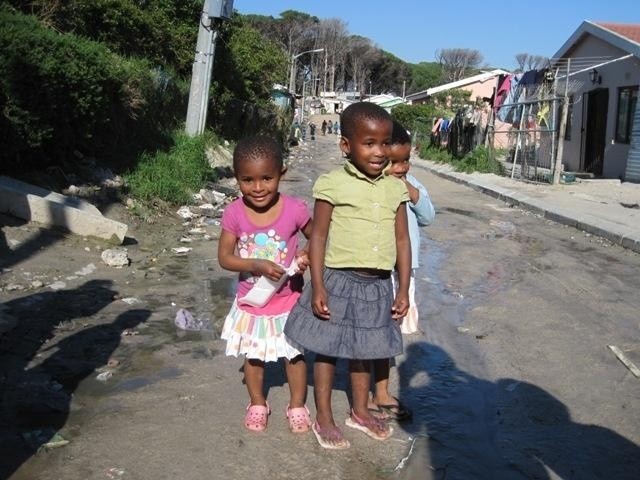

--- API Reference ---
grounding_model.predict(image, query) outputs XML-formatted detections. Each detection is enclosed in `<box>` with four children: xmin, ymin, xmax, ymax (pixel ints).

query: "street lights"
<box><xmin>368</xmin><ymin>81</ymin><xmax>372</xmax><ymax>102</ymax></box>
<box><xmin>301</xmin><ymin>78</ymin><xmax>321</xmax><ymax>123</ymax></box>
<box><xmin>289</xmin><ymin>49</ymin><xmax>325</xmax><ymax>106</ymax></box>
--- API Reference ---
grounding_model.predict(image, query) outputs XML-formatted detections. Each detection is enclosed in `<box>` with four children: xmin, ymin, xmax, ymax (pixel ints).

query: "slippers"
<box><xmin>369</xmin><ymin>395</ymin><xmax>412</xmax><ymax>422</ymax></box>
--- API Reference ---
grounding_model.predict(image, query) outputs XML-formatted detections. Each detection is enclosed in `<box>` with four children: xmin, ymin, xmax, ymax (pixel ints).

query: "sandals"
<box><xmin>244</xmin><ymin>400</ymin><xmax>269</xmax><ymax>431</ymax></box>
<box><xmin>345</xmin><ymin>408</ymin><xmax>393</xmax><ymax>441</ymax></box>
<box><xmin>312</xmin><ymin>420</ymin><xmax>350</xmax><ymax>449</ymax></box>
<box><xmin>286</xmin><ymin>404</ymin><xmax>310</xmax><ymax>433</ymax></box>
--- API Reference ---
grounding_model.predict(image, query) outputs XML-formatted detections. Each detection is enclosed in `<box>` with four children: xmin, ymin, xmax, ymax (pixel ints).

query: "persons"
<box><xmin>362</xmin><ymin>118</ymin><xmax>435</xmax><ymax>424</ymax></box>
<box><xmin>281</xmin><ymin>102</ymin><xmax>412</xmax><ymax>449</ymax></box>
<box><xmin>216</xmin><ymin>135</ymin><xmax>316</xmax><ymax>436</ymax></box>
<box><xmin>289</xmin><ymin>119</ymin><xmax>338</xmax><ymax>145</ymax></box>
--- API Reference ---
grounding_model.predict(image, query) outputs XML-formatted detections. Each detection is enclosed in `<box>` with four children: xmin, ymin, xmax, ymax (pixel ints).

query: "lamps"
<box><xmin>588</xmin><ymin>68</ymin><xmax>602</xmax><ymax>85</ymax></box>
